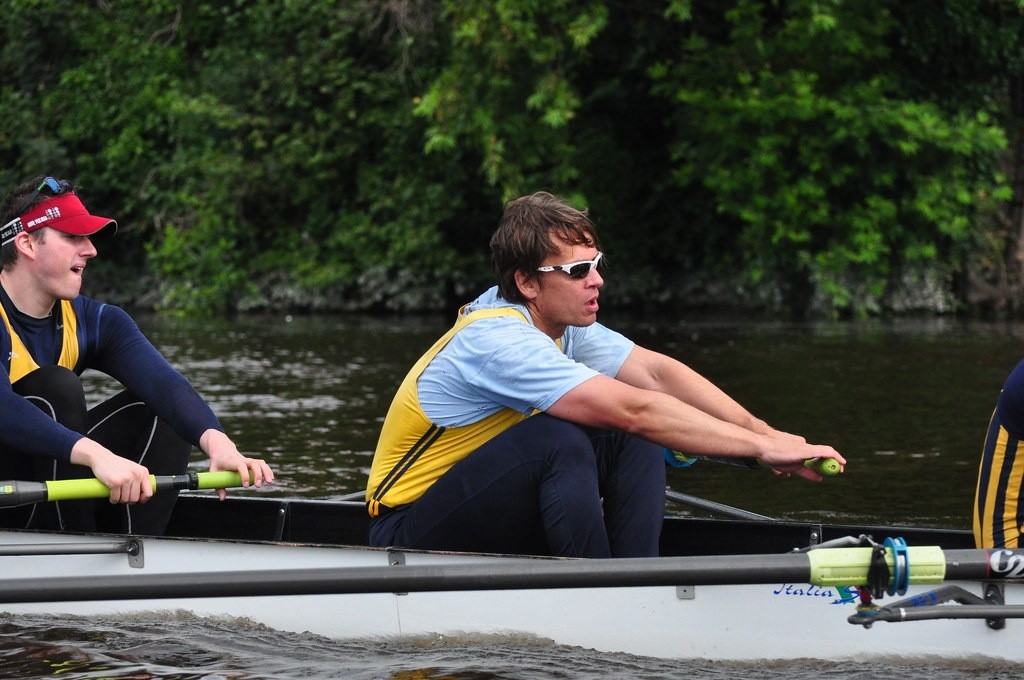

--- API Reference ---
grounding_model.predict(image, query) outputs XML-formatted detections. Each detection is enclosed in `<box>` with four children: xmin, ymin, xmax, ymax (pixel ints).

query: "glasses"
<box><xmin>531</xmin><ymin>251</ymin><xmax>603</xmax><ymax>280</ymax></box>
<box><xmin>9</xmin><ymin>176</ymin><xmax>74</xmax><ymax>223</ymax></box>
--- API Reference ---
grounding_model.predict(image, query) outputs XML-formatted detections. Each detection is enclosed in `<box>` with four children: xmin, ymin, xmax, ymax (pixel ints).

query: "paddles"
<box><xmin>684</xmin><ymin>444</ymin><xmax>842</xmax><ymax>480</ymax></box>
<box><xmin>2</xmin><ymin>533</ymin><xmax>1024</xmax><ymax>612</ymax></box>
<box><xmin>0</xmin><ymin>468</ymin><xmax>268</xmax><ymax>513</ymax></box>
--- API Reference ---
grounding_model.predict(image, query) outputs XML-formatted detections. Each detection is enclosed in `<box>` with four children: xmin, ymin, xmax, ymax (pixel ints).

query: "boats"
<box><xmin>0</xmin><ymin>491</ymin><xmax>1024</xmax><ymax>667</ymax></box>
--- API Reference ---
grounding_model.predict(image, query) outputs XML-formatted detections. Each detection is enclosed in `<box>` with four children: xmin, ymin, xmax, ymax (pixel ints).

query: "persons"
<box><xmin>973</xmin><ymin>361</ymin><xmax>1024</xmax><ymax>549</ymax></box>
<box><xmin>364</xmin><ymin>191</ymin><xmax>847</xmax><ymax>559</ymax></box>
<box><xmin>0</xmin><ymin>174</ymin><xmax>275</xmax><ymax>536</ymax></box>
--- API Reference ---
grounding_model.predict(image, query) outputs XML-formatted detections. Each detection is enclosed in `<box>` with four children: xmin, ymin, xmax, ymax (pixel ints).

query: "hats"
<box><xmin>0</xmin><ymin>192</ymin><xmax>118</xmax><ymax>247</ymax></box>
